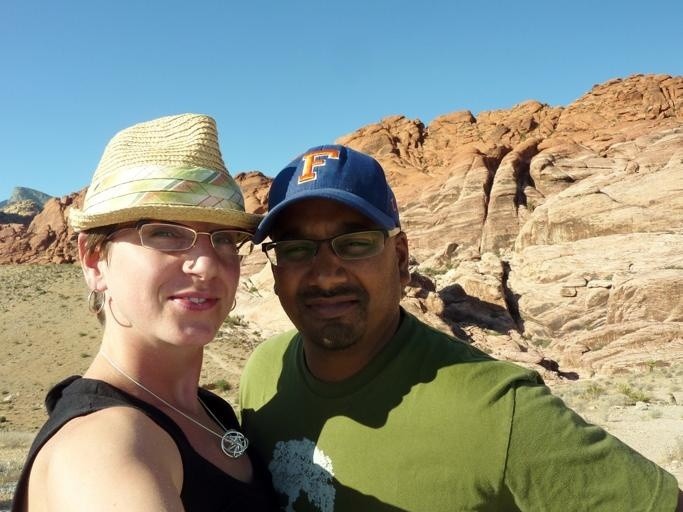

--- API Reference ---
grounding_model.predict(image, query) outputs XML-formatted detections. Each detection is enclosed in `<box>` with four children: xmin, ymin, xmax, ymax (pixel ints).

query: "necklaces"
<box><xmin>99</xmin><ymin>349</ymin><xmax>250</xmax><ymax>460</ymax></box>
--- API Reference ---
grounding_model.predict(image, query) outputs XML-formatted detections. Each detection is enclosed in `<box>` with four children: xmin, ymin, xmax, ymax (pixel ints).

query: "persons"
<box><xmin>10</xmin><ymin>113</ymin><xmax>289</xmax><ymax>511</ymax></box>
<box><xmin>240</xmin><ymin>145</ymin><xmax>683</xmax><ymax>512</ymax></box>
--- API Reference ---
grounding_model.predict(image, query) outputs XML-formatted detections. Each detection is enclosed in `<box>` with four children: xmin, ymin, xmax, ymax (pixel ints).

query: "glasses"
<box><xmin>104</xmin><ymin>223</ymin><xmax>255</xmax><ymax>256</ymax></box>
<box><xmin>262</xmin><ymin>227</ymin><xmax>401</xmax><ymax>270</ymax></box>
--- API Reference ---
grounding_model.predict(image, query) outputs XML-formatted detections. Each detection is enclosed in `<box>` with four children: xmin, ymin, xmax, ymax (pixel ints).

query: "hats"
<box><xmin>68</xmin><ymin>113</ymin><xmax>265</xmax><ymax>230</ymax></box>
<box><xmin>254</xmin><ymin>144</ymin><xmax>401</xmax><ymax>243</ymax></box>
